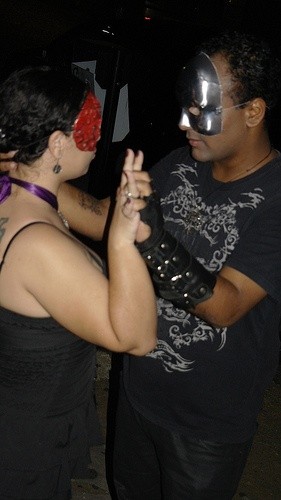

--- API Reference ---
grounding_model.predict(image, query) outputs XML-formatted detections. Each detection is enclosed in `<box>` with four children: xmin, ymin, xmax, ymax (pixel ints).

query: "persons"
<box><xmin>1</xmin><ymin>28</ymin><xmax>281</xmax><ymax>500</ymax></box>
<box><xmin>1</xmin><ymin>65</ymin><xmax>158</xmax><ymax>500</ymax></box>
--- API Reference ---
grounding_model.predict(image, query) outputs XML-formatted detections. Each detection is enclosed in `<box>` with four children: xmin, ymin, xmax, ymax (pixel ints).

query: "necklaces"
<box><xmin>219</xmin><ymin>145</ymin><xmax>273</xmax><ymax>182</ymax></box>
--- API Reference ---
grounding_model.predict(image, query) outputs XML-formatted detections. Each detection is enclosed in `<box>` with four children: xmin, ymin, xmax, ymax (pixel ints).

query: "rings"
<box><xmin>127</xmin><ymin>193</ymin><xmax>136</xmax><ymax>207</ymax></box>
